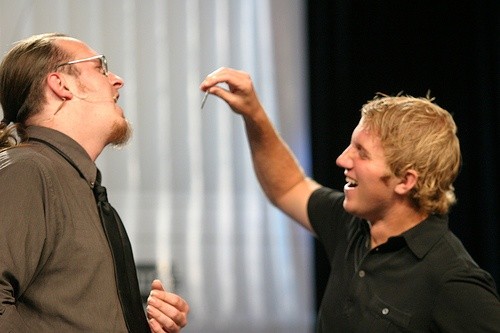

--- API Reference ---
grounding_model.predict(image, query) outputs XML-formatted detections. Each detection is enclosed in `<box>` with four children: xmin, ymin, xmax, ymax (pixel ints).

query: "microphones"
<box><xmin>82</xmin><ymin>96</ymin><xmax>116</xmax><ymax>103</ymax></box>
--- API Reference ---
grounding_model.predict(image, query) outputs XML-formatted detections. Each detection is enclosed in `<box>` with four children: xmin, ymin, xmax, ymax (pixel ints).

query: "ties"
<box><xmin>92</xmin><ymin>168</ymin><xmax>151</xmax><ymax>333</ymax></box>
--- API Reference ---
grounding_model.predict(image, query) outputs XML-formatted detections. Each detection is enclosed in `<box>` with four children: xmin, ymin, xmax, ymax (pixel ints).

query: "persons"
<box><xmin>200</xmin><ymin>67</ymin><xmax>500</xmax><ymax>333</ymax></box>
<box><xmin>0</xmin><ymin>33</ymin><xmax>189</xmax><ymax>333</ymax></box>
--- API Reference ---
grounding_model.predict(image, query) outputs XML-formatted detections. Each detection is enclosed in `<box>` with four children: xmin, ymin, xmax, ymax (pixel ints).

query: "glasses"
<box><xmin>55</xmin><ymin>54</ymin><xmax>108</xmax><ymax>77</ymax></box>
